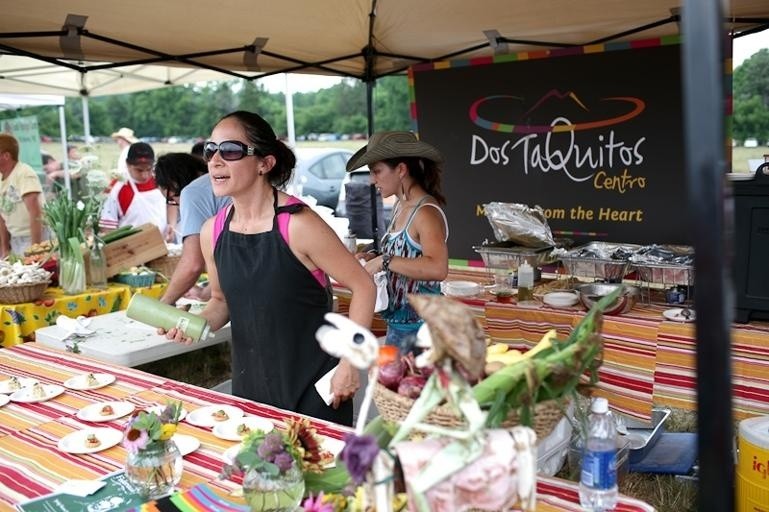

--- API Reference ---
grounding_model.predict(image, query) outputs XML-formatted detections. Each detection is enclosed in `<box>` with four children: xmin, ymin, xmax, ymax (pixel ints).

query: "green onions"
<box><xmin>39</xmin><ymin>179</ymin><xmax>106</xmax><ymax>294</ymax></box>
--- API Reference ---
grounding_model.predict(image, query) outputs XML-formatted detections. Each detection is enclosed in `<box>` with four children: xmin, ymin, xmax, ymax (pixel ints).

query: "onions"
<box><xmin>375</xmin><ymin>358</ymin><xmax>434</xmax><ymax>398</ymax></box>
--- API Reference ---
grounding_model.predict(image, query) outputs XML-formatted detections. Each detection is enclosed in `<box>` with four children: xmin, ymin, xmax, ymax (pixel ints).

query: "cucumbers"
<box><xmin>102</xmin><ymin>223</ymin><xmax>143</xmax><ymax>246</ymax></box>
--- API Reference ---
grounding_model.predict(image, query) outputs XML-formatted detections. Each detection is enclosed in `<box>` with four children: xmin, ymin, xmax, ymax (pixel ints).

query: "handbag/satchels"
<box><xmin>358</xmin><ymin>258</ymin><xmax>389</xmax><ymax>314</ymax></box>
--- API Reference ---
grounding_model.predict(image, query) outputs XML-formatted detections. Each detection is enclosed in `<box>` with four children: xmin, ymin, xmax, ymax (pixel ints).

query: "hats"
<box><xmin>112</xmin><ymin>127</ymin><xmax>140</xmax><ymax>144</ymax></box>
<box><xmin>346</xmin><ymin>131</ymin><xmax>445</xmax><ymax>172</ymax></box>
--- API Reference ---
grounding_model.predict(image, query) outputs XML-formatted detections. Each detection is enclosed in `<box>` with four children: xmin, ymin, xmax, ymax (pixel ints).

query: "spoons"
<box><xmin>682</xmin><ymin>307</ymin><xmax>690</xmax><ymax>322</ymax></box>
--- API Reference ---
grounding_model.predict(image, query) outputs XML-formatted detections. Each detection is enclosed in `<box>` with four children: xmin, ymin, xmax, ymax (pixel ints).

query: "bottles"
<box><xmin>577</xmin><ymin>394</ymin><xmax>619</xmax><ymax>510</ymax></box>
<box><xmin>124</xmin><ymin>291</ymin><xmax>216</xmax><ymax>347</ymax></box>
<box><xmin>126</xmin><ymin>441</ymin><xmax>184</xmax><ymax>498</ymax></box>
<box><xmin>516</xmin><ymin>259</ymin><xmax>534</xmax><ymax>304</ymax></box>
<box><xmin>242</xmin><ymin>465</ymin><xmax>305</xmax><ymax>512</ymax></box>
<box><xmin>343</xmin><ymin>234</ymin><xmax>357</xmax><ymax>254</ymax></box>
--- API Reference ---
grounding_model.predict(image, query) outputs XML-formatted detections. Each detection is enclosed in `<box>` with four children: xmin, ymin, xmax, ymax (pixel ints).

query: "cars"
<box><xmin>290</xmin><ymin>146</ymin><xmax>370</xmax><ymax>210</ymax></box>
<box><xmin>40</xmin><ymin>133</ymin><xmax>203</xmax><ymax>145</ymax></box>
<box><xmin>743</xmin><ymin>137</ymin><xmax>759</xmax><ymax>148</ymax></box>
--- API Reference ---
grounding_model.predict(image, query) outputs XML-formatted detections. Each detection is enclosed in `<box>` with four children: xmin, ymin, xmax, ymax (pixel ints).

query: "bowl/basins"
<box><xmin>574</xmin><ymin>283</ymin><xmax>642</xmax><ymax>317</ymax></box>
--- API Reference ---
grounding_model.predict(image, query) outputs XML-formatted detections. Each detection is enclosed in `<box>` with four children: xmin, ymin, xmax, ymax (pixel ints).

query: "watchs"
<box><xmin>380</xmin><ymin>254</ymin><xmax>391</xmax><ymax>270</ymax></box>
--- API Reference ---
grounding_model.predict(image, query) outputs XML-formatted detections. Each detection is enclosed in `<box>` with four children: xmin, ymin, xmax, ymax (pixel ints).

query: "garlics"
<box><xmin>0</xmin><ymin>259</ymin><xmax>50</xmax><ymax>287</ymax></box>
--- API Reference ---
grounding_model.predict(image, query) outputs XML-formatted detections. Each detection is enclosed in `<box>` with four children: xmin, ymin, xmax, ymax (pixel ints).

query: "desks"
<box><xmin>0</xmin><ymin>258</ymin><xmax>768</xmax><ymax>512</ymax></box>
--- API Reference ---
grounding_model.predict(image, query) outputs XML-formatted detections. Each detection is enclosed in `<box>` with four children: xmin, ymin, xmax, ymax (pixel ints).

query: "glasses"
<box><xmin>202</xmin><ymin>140</ymin><xmax>258</xmax><ymax>161</ymax></box>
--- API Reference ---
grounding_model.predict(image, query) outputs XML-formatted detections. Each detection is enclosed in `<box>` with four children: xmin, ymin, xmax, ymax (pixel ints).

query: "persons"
<box><xmin>158</xmin><ymin>110</ymin><xmax>378</xmax><ymax>428</ymax></box>
<box><xmin>358</xmin><ymin>128</ymin><xmax>449</xmax><ymax>351</ymax></box>
<box><xmin>37</xmin><ymin>127</ymin><xmax>167</xmax><ymax>232</ymax></box>
<box><xmin>150</xmin><ymin>153</ymin><xmax>233</xmax><ymax>336</ymax></box>
<box><xmin>1</xmin><ymin>134</ymin><xmax>59</xmax><ymax>258</ymax></box>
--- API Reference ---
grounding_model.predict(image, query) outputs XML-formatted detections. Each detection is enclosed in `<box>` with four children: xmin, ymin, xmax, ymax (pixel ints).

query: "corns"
<box><xmin>470</xmin><ymin>287</ymin><xmax>625</xmax><ymax>427</ymax></box>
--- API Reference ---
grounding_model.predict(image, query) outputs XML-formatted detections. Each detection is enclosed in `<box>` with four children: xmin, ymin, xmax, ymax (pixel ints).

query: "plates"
<box><xmin>186</xmin><ymin>404</ymin><xmax>246</xmax><ymax>427</ymax></box>
<box><xmin>9</xmin><ymin>384</ymin><xmax>65</xmax><ymax>403</ymax></box>
<box><xmin>76</xmin><ymin>401</ymin><xmax>135</xmax><ymax>423</ymax></box>
<box><xmin>0</xmin><ymin>394</ymin><xmax>9</xmax><ymax>407</ymax></box>
<box><xmin>213</xmin><ymin>417</ymin><xmax>275</xmax><ymax>441</ymax></box>
<box><xmin>725</xmin><ymin>172</ymin><xmax>754</xmax><ymax>181</ymax></box>
<box><xmin>543</xmin><ymin>291</ymin><xmax>580</xmax><ymax>309</ymax></box>
<box><xmin>171</xmin><ymin>434</ymin><xmax>201</xmax><ymax>456</ymax></box>
<box><xmin>64</xmin><ymin>373</ymin><xmax>116</xmax><ymax>391</ymax></box>
<box><xmin>145</xmin><ymin>404</ymin><xmax>186</xmax><ymax>422</ymax></box>
<box><xmin>662</xmin><ymin>307</ymin><xmax>698</xmax><ymax>323</ymax></box>
<box><xmin>489</xmin><ymin>288</ymin><xmax>517</xmax><ymax>296</ymax></box>
<box><xmin>443</xmin><ymin>278</ymin><xmax>481</xmax><ymax>298</ymax></box>
<box><xmin>288</xmin><ymin>434</ymin><xmax>346</xmax><ymax>471</ymax></box>
<box><xmin>0</xmin><ymin>377</ymin><xmax>40</xmax><ymax>394</ymax></box>
<box><xmin>220</xmin><ymin>441</ymin><xmax>247</xmax><ymax>466</ymax></box>
<box><xmin>57</xmin><ymin>427</ymin><xmax>124</xmax><ymax>455</ymax></box>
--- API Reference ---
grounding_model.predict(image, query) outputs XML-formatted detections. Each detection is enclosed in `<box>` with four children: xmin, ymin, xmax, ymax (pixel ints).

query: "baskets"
<box><xmin>474</xmin><ymin>240</ymin><xmax>696</xmax><ymax>307</ymax></box>
<box><xmin>0</xmin><ymin>280</ymin><xmax>52</xmax><ymax>304</ymax></box>
<box><xmin>369</xmin><ymin>372</ymin><xmax>570</xmax><ymax>444</ymax></box>
<box><xmin>114</xmin><ymin>248</ymin><xmax>182</xmax><ymax>287</ymax></box>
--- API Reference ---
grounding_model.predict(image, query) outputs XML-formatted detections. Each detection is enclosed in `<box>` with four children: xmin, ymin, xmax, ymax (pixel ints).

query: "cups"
<box><xmin>747</xmin><ymin>158</ymin><xmax>768</xmax><ymax>173</ymax></box>
<box><xmin>493</xmin><ymin>272</ymin><xmax>514</xmax><ymax>304</ymax></box>
